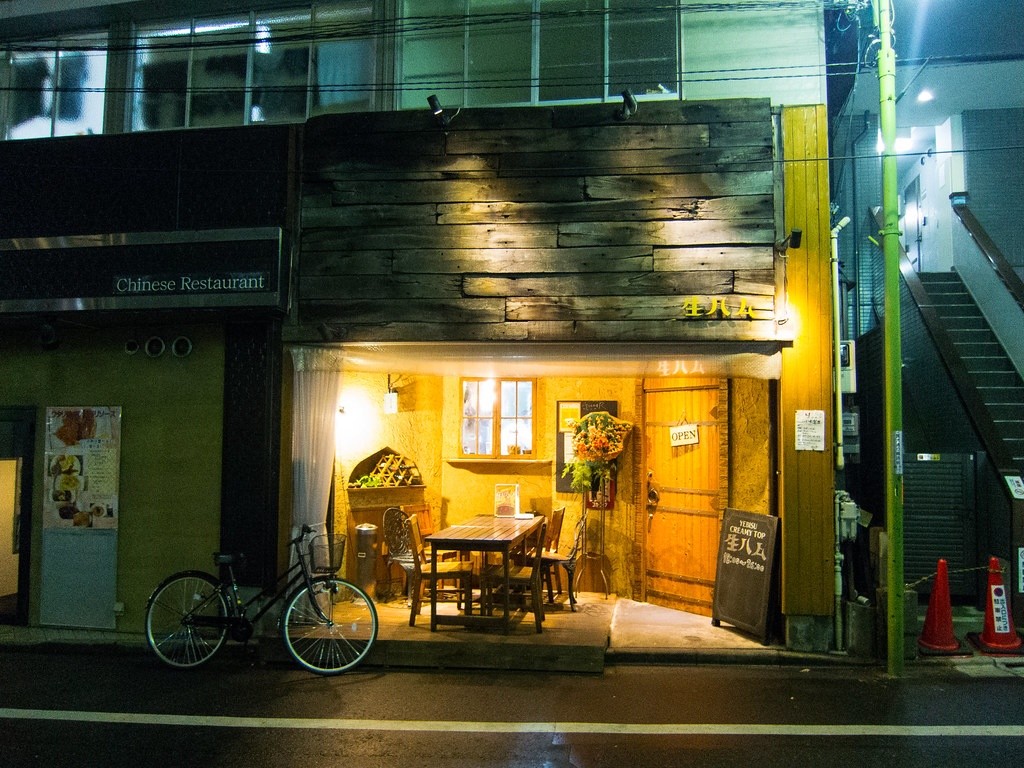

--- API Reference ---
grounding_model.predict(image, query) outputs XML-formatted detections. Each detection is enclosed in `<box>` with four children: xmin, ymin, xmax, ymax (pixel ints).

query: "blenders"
<box><xmin>480</xmin><ymin>421</ymin><xmax>491</xmax><ymax>454</ymax></box>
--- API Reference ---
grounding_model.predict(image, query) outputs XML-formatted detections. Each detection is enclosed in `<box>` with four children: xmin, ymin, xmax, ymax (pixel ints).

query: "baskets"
<box><xmin>308</xmin><ymin>534</ymin><xmax>347</xmax><ymax>574</ymax></box>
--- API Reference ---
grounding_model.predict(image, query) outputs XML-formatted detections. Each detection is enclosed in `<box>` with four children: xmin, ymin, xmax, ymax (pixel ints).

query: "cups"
<box><xmin>523</xmin><ymin>449</ymin><xmax>531</xmax><ymax>454</ymax></box>
<box><xmin>858</xmin><ymin>596</ymin><xmax>871</xmax><ymax>606</ymax></box>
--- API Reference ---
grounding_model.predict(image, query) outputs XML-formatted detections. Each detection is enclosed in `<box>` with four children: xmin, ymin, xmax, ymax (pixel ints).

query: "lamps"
<box><xmin>776</xmin><ymin>227</ymin><xmax>802</xmax><ymax>257</ymax></box>
<box><xmin>427</xmin><ymin>93</ymin><xmax>452</xmax><ymax>126</ymax></box>
<box><xmin>615</xmin><ymin>88</ymin><xmax>638</xmax><ymax>120</ymax></box>
<box><xmin>384</xmin><ymin>386</ymin><xmax>399</xmax><ymax>416</ymax></box>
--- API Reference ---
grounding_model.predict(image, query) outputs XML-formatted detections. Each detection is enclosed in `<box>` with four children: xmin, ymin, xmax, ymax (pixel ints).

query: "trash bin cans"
<box><xmin>351</xmin><ymin>523</ymin><xmax>380</xmax><ymax>604</ymax></box>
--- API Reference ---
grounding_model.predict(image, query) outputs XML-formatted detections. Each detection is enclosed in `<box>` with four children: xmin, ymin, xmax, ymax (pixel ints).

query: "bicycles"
<box><xmin>144</xmin><ymin>524</ymin><xmax>381</xmax><ymax>677</ymax></box>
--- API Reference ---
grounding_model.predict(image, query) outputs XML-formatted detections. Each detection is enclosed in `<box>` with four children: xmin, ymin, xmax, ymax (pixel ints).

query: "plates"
<box><xmin>53</xmin><ymin>501</ymin><xmax>83</xmax><ymax>522</ymax></box>
<box><xmin>54</xmin><ymin>474</ymin><xmax>86</xmax><ymax>490</ymax></box>
<box><xmin>74</xmin><ymin>512</ymin><xmax>92</xmax><ymax>528</ymax></box>
<box><xmin>91</xmin><ymin>502</ymin><xmax>107</xmax><ymax>518</ymax></box>
<box><xmin>48</xmin><ymin>488</ymin><xmax>76</xmax><ymax>502</ymax></box>
<box><xmin>50</xmin><ymin>454</ymin><xmax>81</xmax><ymax>476</ymax></box>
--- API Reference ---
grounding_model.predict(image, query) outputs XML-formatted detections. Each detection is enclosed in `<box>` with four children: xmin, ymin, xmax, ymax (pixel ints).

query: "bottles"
<box><xmin>507</xmin><ymin>431</ymin><xmax>521</xmax><ymax>454</ymax></box>
<box><xmin>379</xmin><ymin>462</ymin><xmax>414</xmax><ymax>486</ymax></box>
<box><xmin>464</xmin><ymin>446</ymin><xmax>470</xmax><ymax>455</ymax></box>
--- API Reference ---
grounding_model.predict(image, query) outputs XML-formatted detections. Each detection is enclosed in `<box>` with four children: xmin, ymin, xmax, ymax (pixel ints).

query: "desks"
<box><xmin>424</xmin><ymin>513</ymin><xmax>545</xmax><ymax>632</ymax></box>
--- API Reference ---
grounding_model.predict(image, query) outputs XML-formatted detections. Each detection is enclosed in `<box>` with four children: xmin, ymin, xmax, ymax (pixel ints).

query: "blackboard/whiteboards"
<box><xmin>713</xmin><ymin>507</ymin><xmax>782</xmax><ymax>637</ymax></box>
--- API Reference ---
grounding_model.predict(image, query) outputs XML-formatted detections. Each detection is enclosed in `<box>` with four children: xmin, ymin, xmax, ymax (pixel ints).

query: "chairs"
<box><xmin>405</xmin><ymin>513</ymin><xmax>475</xmax><ymax>632</ymax></box>
<box><xmin>511</xmin><ymin>505</ymin><xmax>566</xmax><ymax>602</ymax></box>
<box><xmin>480</xmin><ymin>515</ymin><xmax>550</xmax><ymax>633</ymax></box>
<box><xmin>384</xmin><ymin>507</ymin><xmax>431</xmax><ymax>599</ymax></box>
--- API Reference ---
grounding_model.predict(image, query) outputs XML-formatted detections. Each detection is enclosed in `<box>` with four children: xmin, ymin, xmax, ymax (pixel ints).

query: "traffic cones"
<box><xmin>979</xmin><ymin>556</ymin><xmax>1021</xmax><ymax>651</ymax></box>
<box><xmin>919</xmin><ymin>558</ymin><xmax>960</xmax><ymax>651</ymax></box>
